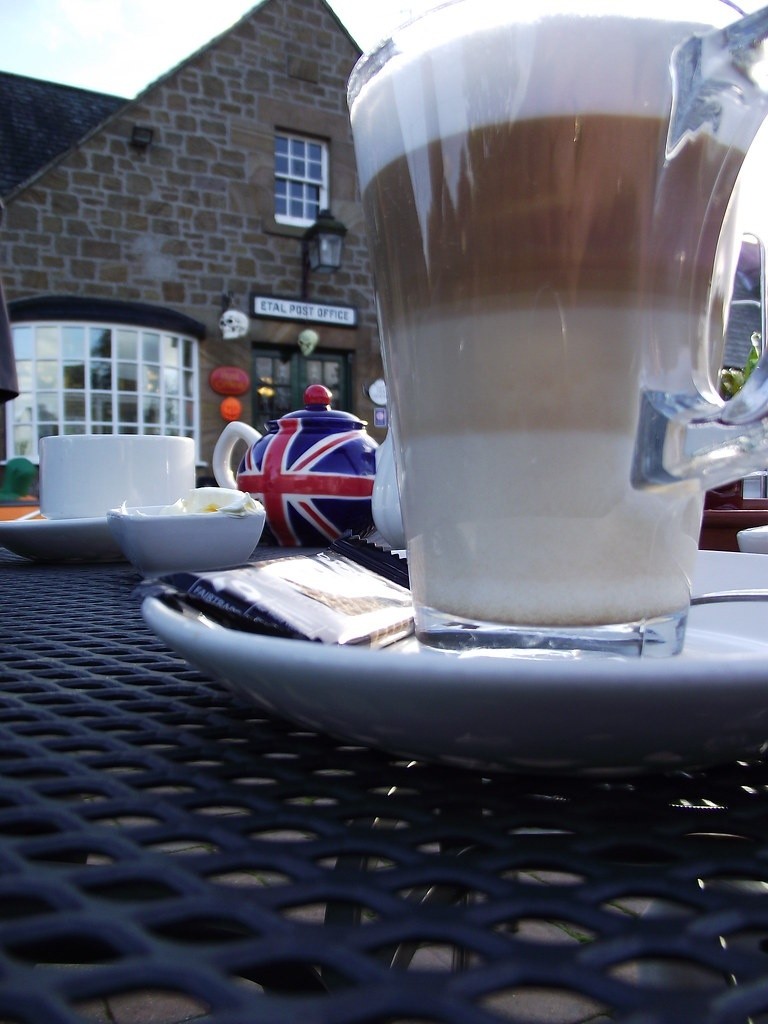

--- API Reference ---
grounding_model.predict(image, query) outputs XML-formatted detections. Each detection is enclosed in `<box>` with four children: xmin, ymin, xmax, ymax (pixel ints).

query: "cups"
<box><xmin>36</xmin><ymin>433</ymin><xmax>199</xmax><ymax>519</ymax></box>
<box><xmin>345</xmin><ymin>0</ymin><xmax>768</xmax><ymax>659</ymax></box>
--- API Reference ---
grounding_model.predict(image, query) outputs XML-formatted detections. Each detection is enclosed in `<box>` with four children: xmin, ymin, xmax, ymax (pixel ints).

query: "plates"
<box><xmin>146</xmin><ymin>547</ymin><xmax>766</xmax><ymax>763</ymax></box>
<box><xmin>1</xmin><ymin>487</ymin><xmax>267</xmax><ymax>574</ymax></box>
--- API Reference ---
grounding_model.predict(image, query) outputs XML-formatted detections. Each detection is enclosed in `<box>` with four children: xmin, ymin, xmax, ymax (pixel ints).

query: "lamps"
<box><xmin>300</xmin><ymin>209</ymin><xmax>347</xmax><ymax>299</ymax></box>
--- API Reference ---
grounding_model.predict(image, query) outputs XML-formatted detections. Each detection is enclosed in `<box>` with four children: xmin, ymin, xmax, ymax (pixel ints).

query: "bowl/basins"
<box><xmin>106</xmin><ymin>503</ymin><xmax>266</xmax><ymax>580</ymax></box>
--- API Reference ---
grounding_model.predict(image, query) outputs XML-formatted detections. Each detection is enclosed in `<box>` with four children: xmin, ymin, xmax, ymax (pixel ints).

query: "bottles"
<box><xmin>371</xmin><ymin>424</ymin><xmax>408</xmax><ymax>546</ymax></box>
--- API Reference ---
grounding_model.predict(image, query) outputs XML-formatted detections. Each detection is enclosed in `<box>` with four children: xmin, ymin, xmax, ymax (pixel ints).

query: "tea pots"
<box><xmin>212</xmin><ymin>387</ymin><xmax>379</xmax><ymax>548</ymax></box>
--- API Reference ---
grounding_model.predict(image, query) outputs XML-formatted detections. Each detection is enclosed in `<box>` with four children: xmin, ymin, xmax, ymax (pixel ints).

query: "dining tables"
<box><xmin>0</xmin><ymin>524</ymin><xmax>765</xmax><ymax>1023</ymax></box>
